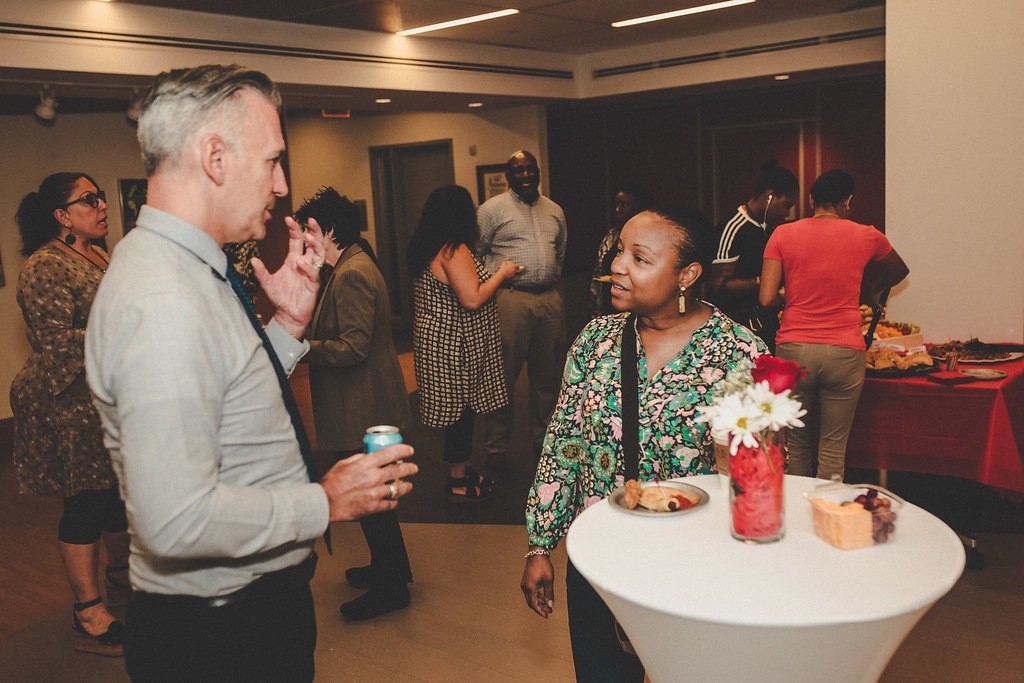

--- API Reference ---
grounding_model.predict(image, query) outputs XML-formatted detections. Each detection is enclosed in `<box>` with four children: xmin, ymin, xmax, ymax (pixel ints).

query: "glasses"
<box><xmin>61</xmin><ymin>191</ymin><xmax>106</xmax><ymax>209</ymax></box>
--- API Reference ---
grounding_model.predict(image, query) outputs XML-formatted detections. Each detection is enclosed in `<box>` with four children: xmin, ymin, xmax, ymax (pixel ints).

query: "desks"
<box><xmin>567</xmin><ymin>475</ymin><xmax>965</xmax><ymax>683</ymax></box>
<box><xmin>842</xmin><ymin>343</ymin><xmax>1024</xmax><ymax>497</ymax></box>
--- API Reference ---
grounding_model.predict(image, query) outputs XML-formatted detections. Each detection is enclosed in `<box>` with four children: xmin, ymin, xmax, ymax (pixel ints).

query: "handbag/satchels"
<box><xmin>720</xmin><ymin>277</ymin><xmax>774</xmax><ymax>349</ymax></box>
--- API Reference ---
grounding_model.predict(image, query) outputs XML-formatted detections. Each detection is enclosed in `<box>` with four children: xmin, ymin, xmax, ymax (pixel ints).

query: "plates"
<box><xmin>608</xmin><ymin>481</ymin><xmax>710</xmax><ymax>517</ymax></box>
<box><xmin>959</xmin><ymin>369</ymin><xmax>1008</xmax><ymax>380</ymax></box>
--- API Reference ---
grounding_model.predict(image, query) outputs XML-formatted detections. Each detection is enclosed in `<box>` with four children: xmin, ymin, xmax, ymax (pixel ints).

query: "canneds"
<box><xmin>362</xmin><ymin>425</ymin><xmax>403</xmax><ymax>485</ymax></box>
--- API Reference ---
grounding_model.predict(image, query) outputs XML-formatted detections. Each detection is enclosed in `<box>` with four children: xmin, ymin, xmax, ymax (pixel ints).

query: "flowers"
<box><xmin>694</xmin><ymin>349</ymin><xmax>810</xmax><ymax>453</ymax></box>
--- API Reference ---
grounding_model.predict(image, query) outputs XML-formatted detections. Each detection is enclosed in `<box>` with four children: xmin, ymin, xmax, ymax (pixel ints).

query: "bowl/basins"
<box><xmin>802</xmin><ymin>479</ymin><xmax>906</xmax><ymax>551</ymax></box>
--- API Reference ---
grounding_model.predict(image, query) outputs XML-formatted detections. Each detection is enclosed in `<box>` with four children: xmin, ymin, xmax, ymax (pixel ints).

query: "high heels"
<box><xmin>105</xmin><ymin>564</ymin><xmax>130</xmax><ymax>607</ymax></box>
<box><xmin>71</xmin><ymin>597</ymin><xmax>126</xmax><ymax>656</ymax></box>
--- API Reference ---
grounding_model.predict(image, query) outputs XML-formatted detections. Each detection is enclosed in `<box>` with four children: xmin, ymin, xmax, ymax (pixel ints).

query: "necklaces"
<box><xmin>813</xmin><ymin>213</ymin><xmax>840</xmax><ymax>218</ymax></box>
<box><xmin>55</xmin><ymin>237</ymin><xmax>109</xmax><ymax>269</ymax></box>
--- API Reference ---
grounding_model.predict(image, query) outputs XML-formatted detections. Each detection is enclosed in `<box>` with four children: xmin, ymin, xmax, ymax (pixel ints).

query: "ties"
<box><xmin>226</xmin><ymin>261</ymin><xmax>334</xmax><ymax>556</ymax></box>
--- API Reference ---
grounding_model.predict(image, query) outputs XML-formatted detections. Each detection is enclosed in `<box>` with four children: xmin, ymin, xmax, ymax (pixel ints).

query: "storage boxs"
<box><xmin>807</xmin><ymin>482</ymin><xmax>904</xmax><ymax>544</ymax></box>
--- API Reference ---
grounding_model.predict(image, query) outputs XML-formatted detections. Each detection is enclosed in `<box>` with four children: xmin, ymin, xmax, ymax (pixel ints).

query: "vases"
<box><xmin>730</xmin><ymin>442</ymin><xmax>786</xmax><ymax>543</ymax></box>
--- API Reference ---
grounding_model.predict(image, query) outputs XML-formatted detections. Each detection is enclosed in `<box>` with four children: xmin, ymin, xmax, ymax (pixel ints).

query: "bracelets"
<box><xmin>524</xmin><ymin>549</ymin><xmax>550</xmax><ymax>559</ymax></box>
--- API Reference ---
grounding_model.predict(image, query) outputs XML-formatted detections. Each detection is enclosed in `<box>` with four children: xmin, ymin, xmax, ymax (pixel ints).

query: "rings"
<box><xmin>390</xmin><ymin>484</ymin><xmax>397</xmax><ymax>499</ymax></box>
<box><xmin>315</xmin><ymin>263</ymin><xmax>323</xmax><ymax>268</ymax></box>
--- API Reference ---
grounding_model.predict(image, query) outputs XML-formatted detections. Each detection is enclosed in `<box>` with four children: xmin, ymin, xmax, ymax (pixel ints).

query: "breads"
<box><xmin>865</xmin><ymin>346</ymin><xmax>933</xmax><ymax>369</ymax></box>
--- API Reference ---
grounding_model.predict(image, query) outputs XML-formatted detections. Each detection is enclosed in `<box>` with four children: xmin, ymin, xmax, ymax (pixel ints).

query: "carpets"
<box><xmin>312</xmin><ymin>359</ymin><xmax>537</xmax><ymax>527</ymax></box>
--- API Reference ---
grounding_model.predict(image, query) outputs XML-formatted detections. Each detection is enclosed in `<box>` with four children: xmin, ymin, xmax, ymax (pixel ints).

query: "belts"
<box><xmin>209</xmin><ymin>555</ymin><xmax>318</xmax><ymax>607</ymax></box>
<box><xmin>500</xmin><ymin>283</ymin><xmax>552</xmax><ymax>294</ymax></box>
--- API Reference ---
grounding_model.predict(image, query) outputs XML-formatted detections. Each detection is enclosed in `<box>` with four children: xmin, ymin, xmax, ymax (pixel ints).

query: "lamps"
<box><xmin>126</xmin><ymin>90</ymin><xmax>145</xmax><ymax>122</ymax></box>
<box><xmin>37</xmin><ymin>84</ymin><xmax>58</xmax><ymax>121</ymax></box>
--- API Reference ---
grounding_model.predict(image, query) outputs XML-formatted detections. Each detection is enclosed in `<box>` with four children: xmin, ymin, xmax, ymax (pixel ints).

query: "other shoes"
<box><xmin>341</xmin><ymin>581</ymin><xmax>410</xmax><ymax>625</ymax></box>
<box><xmin>345</xmin><ymin>565</ymin><xmax>412</xmax><ymax>587</ymax></box>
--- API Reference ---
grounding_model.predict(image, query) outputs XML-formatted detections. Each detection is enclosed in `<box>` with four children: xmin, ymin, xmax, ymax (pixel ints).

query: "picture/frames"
<box><xmin>476</xmin><ymin>163</ymin><xmax>509</xmax><ymax>205</ymax></box>
<box><xmin>116</xmin><ymin>178</ymin><xmax>147</xmax><ymax>238</ymax></box>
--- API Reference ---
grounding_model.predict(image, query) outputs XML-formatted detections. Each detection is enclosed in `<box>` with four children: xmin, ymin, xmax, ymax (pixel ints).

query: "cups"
<box><xmin>713</xmin><ymin>438</ymin><xmax>730</xmax><ymax>496</ymax></box>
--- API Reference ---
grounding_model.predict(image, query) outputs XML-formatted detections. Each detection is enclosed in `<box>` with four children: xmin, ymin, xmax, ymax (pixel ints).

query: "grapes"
<box><xmin>841</xmin><ymin>494</ymin><xmax>895</xmax><ymax>544</ymax></box>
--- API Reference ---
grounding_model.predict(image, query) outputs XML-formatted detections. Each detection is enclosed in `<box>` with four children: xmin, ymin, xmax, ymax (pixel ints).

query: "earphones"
<box><xmin>768</xmin><ymin>195</ymin><xmax>773</xmax><ymax>204</ymax></box>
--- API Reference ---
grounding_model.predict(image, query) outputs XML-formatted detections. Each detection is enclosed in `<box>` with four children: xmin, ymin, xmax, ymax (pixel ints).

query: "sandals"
<box><xmin>466</xmin><ymin>468</ymin><xmax>495</xmax><ymax>487</ymax></box>
<box><xmin>445</xmin><ymin>477</ymin><xmax>492</xmax><ymax>503</ymax></box>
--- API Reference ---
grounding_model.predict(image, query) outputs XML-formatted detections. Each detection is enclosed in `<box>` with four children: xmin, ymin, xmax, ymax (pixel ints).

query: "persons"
<box><xmin>759</xmin><ymin>169</ymin><xmax>909</xmax><ymax>483</ymax></box>
<box><xmin>295</xmin><ymin>187</ymin><xmax>414</xmax><ymax>621</ymax></box>
<box><xmin>589</xmin><ymin>179</ymin><xmax>650</xmax><ymax>318</ymax></box>
<box><xmin>475</xmin><ymin>150</ymin><xmax>567</xmax><ymax>466</ymax></box>
<box><xmin>8</xmin><ymin>172</ymin><xmax>134</xmax><ymax>648</ymax></box>
<box><xmin>406</xmin><ymin>185</ymin><xmax>519</xmax><ymax>504</ymax></box>
<box><xmin>521</xmin><ymin>201</ymin><xmax>771</xmax><ymax>683</ymax></box>
<box><xmin>85</xmin><ymin>64</ymin><xmax>418</xmax><ymax>683</ymax></box>
<box><xmin>711</xmin><ymin>167</ymin><xmax>800</xmax><ymax>358</ymax></box>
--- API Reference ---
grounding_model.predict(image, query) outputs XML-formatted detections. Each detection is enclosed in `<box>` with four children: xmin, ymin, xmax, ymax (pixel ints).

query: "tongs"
<box><xmin>864</xmin><ymin>287</ymin><xmax>892</xmax><ymax>352</ymax></box>
